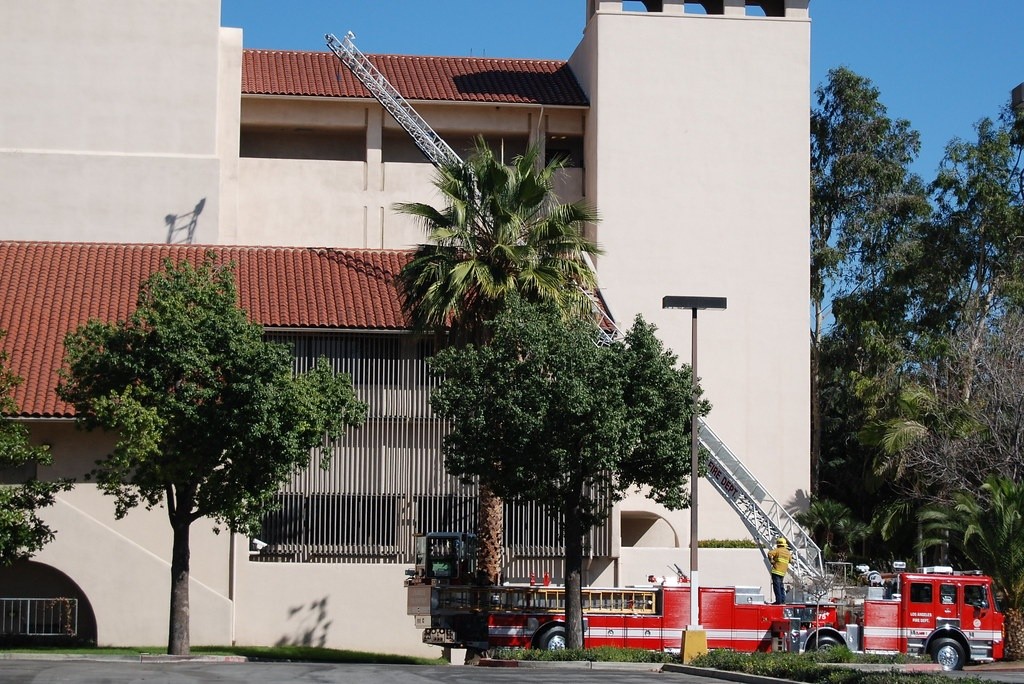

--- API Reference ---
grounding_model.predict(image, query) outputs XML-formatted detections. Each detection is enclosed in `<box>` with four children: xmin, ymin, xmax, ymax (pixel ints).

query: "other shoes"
<box><xmin>771</xmin><ymin>601</ymin><xmax>787</xmax><ymax>606</ymax></box>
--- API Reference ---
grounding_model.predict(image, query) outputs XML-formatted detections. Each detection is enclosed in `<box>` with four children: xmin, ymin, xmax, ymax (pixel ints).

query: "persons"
<box><xmin>768</xmin><ymin>538</ymin><xmax>792</xmax><ymax>604</ymax></box>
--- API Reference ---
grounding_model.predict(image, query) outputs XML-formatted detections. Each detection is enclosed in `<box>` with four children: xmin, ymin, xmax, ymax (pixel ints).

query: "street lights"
<box><xmin>662</xmin><ymin>294</ymin><xmax>729</xmax><ymax>663</ymax></box>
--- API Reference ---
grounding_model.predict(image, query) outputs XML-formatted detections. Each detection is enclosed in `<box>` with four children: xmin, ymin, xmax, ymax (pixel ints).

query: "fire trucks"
<box><xmin>322</xmin><ymin>32</ymin><xmax>1006</xmax><ymax>674</ymax></box>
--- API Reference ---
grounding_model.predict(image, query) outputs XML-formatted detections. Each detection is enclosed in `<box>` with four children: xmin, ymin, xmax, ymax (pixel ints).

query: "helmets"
<box><xmin>776</xmin><ymin>538</ymin><xmax>788</xmax><ymax>545</ymax></box>
